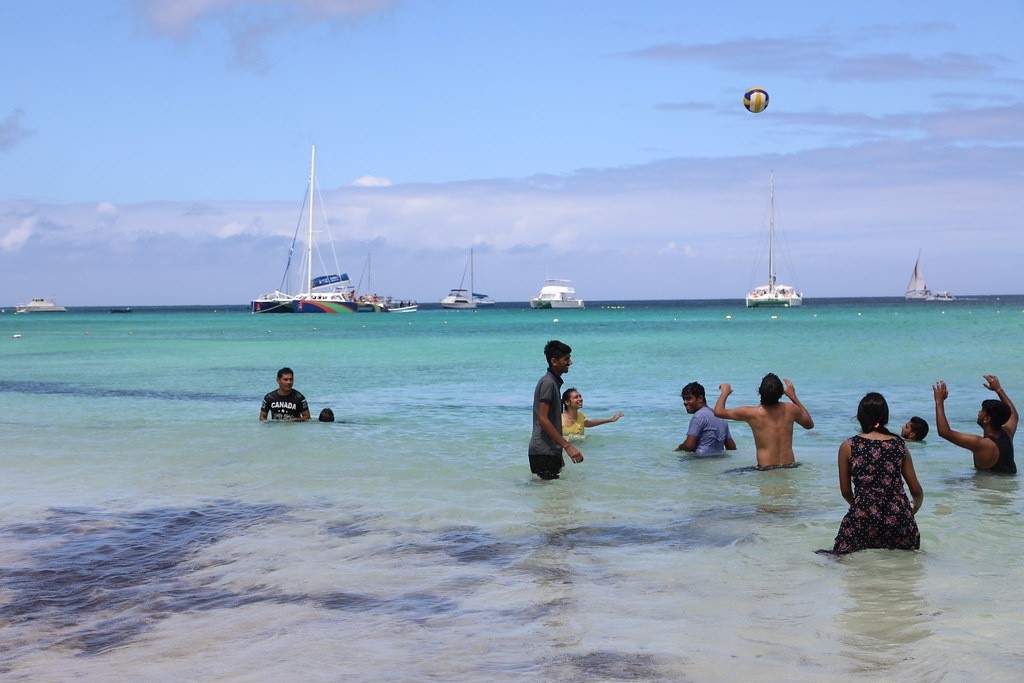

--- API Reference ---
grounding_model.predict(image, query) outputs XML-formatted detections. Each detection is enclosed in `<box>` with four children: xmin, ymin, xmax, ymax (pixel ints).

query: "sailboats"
<box><xmin>746</xmin><ymin>170</ymin><xmax>804</xmax><ymax>308</ymax></box>
<box><xmin>439</xmin><ymin>247</ymin><xmax>477</xmax><ymax>309</ymax></box>
<box><xmin>903</xmin><ymin>247</ymin><xmax>933</xmax><ymax>300</ymax></box>
<box><xmin>250</xmin><ymin>144</ymin><xmax>391</xmax><ymax>314</ymax></box>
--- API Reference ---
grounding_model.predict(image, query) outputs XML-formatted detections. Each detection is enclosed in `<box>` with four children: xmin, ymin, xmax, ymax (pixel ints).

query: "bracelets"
<box><xmin>996</xmin><ymin>388</ymin><xmax>1003</xmax><ymax>392</ymax></box>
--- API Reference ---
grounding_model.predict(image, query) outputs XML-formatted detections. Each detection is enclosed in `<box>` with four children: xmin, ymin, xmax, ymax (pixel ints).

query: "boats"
<box><xmin>529</xmin><ymin>278</ymin><xmax>585</xmax><ymax>310</ymax></box>
<box><xmin>387</xmin><ymin>304</ymin><xmax>419</xmax><ymax>314</ymax></box>
<box><xmin>110</xmin><ymin>307</ymin><xmax>133</xmax><ymax>313</ymax></box>
<box><xmin>16</xmin><ymin>297</ymin><xmax>68</xmax><ymax>313</ymax></box>
<box><xmin>924</xmin><ymin>291</ymin><xmax>959</xmax><ymax>302</ymax></box>
<box><xmin>471</xmin><ymin>293</ymin><xmax>496</xmax><ymax>305</ymax></box>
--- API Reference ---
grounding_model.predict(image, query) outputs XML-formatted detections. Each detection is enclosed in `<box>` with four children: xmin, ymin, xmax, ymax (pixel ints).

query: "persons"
<box><xmin>834</xmin><ymin>392</ymin><xmax>924</xmax><ymax>556</ymax></box>
<box><xmin>932</xmin><ymin>375</ymin><xmax>1019</xmax><ymax>474</ymax></box>
<box><xmin>901</xmin><ymin>416</ymin><xmax>929</xmax><ymax>441</ymax></box>
<box><xmin>780</xmin><ymin>289</ymin><xmax>785</xmax><ymax>294</ymax></box>
<box><xmin>350</xmin><ymin>290</ymin><xmax>410</xmax><ymax>308</ymax></box>
<box><xmin>528</xmin><ymin>340</ymin><xmax>584</xmax><ymax>481</ymax></box>
<box><xmin>753</xmin><ymin>289</ymin><xmax>767</xmax><ymax>295</ymax></box>
<box><xmin>319</xmin><ymin>407</ymin><xmax>335</xmax><ymax>422</ymax></box>
<box><xmin>714</xmin><ymin>373</ymin><xmax>814</xmax><ymax>467</ymax></box>
<box><xmin>675</xmin><ymin>381</ymin><xmax>737</xmax><ymax>456</ymax></box>
<box><xmin>259</xmin><ymin>367</ymin><xmax>310</xmax><ymax>422</ymax></box>
<box><xmin>561</xmin><ymin>388</ymin><xmax>625</xmax><ymax>436</ymax></box>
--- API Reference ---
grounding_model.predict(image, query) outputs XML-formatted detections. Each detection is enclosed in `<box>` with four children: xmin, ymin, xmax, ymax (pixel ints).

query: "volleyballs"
<box><xmin>743</xmin><ymin>85</ymin><xmax>770</xmax><ymax>115</ymax></box>
